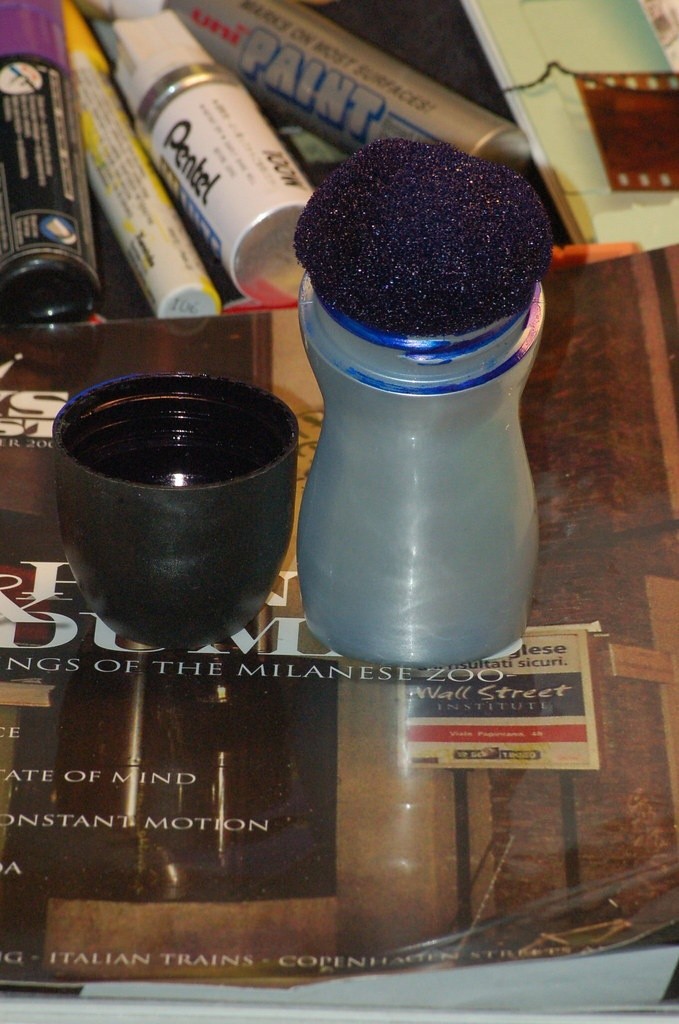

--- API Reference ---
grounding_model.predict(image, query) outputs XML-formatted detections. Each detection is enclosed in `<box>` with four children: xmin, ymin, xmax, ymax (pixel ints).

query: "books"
<box><xmin>1</xmin><ymin>243</ymin><xmax>679</xmax><ymax>1023</ymax></box>
<box><xmin>460</xmin><ymin>0</ymin><xmax>678</xmax><ymax>260</ymax></box>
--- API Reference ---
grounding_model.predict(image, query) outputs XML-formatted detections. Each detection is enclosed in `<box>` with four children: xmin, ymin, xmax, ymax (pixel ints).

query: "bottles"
<box><xmin>0</xmin><ymin>0</ymin><xmax>103</xmax><ymax>321</ymax></box>
<box><xmin>299</xmin><ymin>140</ymin><xmax>545</xmax><ymax>671</ymax></box>
<box><xmin>112</xmin><ymin>9</ymin><xmax>326</xmax><ymax>306</ymax></box>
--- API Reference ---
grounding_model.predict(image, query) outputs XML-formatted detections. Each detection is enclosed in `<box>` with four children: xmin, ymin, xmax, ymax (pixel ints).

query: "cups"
<box><xmin>46</xmin><ymin>372</ymin><xmax>301</xmax><ymax>647</ymax></box>
